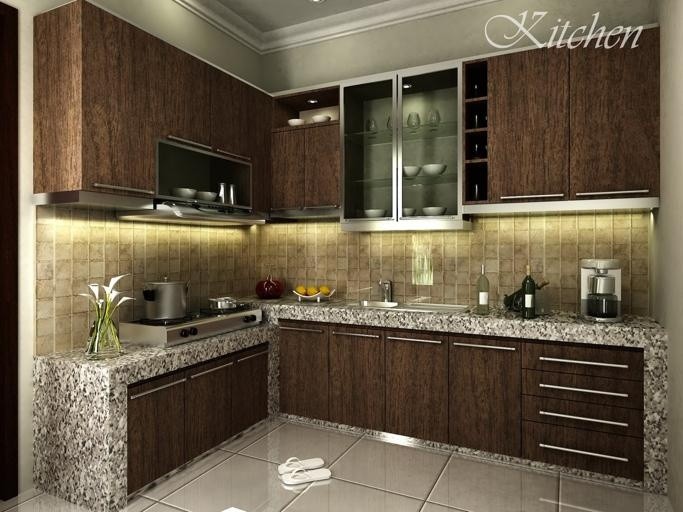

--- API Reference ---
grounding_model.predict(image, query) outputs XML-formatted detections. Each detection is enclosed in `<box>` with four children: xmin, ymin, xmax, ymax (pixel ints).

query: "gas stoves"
<box><xmin>118</xmin><ymin>306</ymin><xmax>262</xmax><ymax>347</ymax></box>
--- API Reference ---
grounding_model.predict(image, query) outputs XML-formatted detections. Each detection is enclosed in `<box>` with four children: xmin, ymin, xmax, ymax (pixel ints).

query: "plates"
<box><xmin>292</xmin><ymin>289</ymin><xmax>335</xmax><ymax>303</ymax></box>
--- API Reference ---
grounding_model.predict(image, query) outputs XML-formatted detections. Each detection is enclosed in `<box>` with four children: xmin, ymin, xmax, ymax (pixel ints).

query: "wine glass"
<box><xmin>427</xmin><ymin>110</ymin><xmax>440</xmax><ymax>132</ymax></box>
<box><xmin>407</xmin><ymin>112</ymin><xmax>421</xmax><ymax>134</ymax></box>
<box><xmin>365</xmin><ymin>118</ymin><xmax>378</xmax><ymax>138</ymax></box>
<box><xmin>386</xmin><ymin>115</ymin><xmax>400</xmax><ymax>137</ymax></box>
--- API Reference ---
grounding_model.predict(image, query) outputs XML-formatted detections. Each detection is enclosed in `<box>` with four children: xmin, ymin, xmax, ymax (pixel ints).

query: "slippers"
<box><xmin>281</xmin><ymin>467</ymin><xmax>331</xmax><ymax>486</ymax></box>
<box><xmin>277</xmin><ymin>456</ymin><xmax>324</xmax><ymax>475</ymax></box>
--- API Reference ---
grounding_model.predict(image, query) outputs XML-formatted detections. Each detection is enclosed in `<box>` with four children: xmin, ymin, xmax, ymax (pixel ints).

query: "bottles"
<box><xmin>217</xmin><ymin>183</ymin><xmax>228</xmax><ymax>203</ymax></box>
<box><xmin>504</xmin><ymin>277</ymin><xmax>550</xmax><ymax>307</ymax></box>
<box><xmin>523</xmin><ymin>265</ymin><xmax>536</xmax><ymax>319</ymax></box>
<box><xmin>476</xmin><ymin>265</ymin><xmax>490</xmax><ymax>315</ymax></box>
<box><xmin>228</xmin><ymin>184</ymin><xmax>237</xmax><ymax>205</ymax></box>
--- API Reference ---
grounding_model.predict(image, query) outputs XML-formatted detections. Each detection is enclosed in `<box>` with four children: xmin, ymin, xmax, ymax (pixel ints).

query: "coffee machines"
<box><xmin>578</xmin><ymin>258</ymin><xmax>623</xmax><ymax>322</ymax></box>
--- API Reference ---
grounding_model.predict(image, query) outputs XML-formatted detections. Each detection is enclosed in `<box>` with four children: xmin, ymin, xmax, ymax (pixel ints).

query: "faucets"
<box><xmin>378</xmin><ymin>279</ymin><xmax>392</xmax><ymax>301</ymax></box>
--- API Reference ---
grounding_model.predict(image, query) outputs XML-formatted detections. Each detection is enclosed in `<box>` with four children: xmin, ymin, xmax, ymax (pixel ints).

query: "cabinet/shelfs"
<box><xmin>125</xmin><ymin>340</ymin><xmax>270</xmax><ymax>495</ymax></box>
<box><xmin>462</xmin><ymin>25</ymin><xmax>660</xmax><ymax>206</ymax></box>
<box><xmin>268</xmin><ymin>120</ymin><xmax>343</xmax><ymax>211</ymax></box>
<box><xmin>277</xmin><ymin>318</ymin><xmax>646</xmax><ymax>485</ymax></box>
<box><xmin>31</xmin><ymin>0</ymin><xmax>270</xmax><ymax>219</ymax></box>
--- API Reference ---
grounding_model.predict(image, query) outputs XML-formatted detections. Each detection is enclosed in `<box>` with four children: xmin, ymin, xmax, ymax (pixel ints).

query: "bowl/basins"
<box><xmin>171</xmin><ymin>188</ymin><xmax>197</xmax><ymax>198</ymax></box>
<box><xmin>422</xmin><ymin>164</ymin><xmax>447</xmax><ymax>175</ymax></box>
<box><xmin>422</xmin><ymin>207</ymin><xmax>447</xmax><ymax>216</ymax></box>
<box><xmin>313</xmin><ymin>115</ymin><xmax>331</xmax><ymax>122</ymax></box>
<box><xmin>288</xmin><ymin>119</ymin><xmax>305</xmax><ymax>126</ymax></box>
<box><xmin>364</xmin><ymin>210</ymin><xmax>387</xmax><ymax>217</ymax></box>
<box><xmin>402</xmin><ymin>165</ymin><xmax>421</xmax><ymax>176</ymax></box>
<box><xmin>403</xmin><ymin>208</ymin><xmax>415</xmax><ymax>216</ymax></box>
<box><xmin>197</xmin><ymin>192</ymin><xmax>217</xmax><ymax>201</ymax></box>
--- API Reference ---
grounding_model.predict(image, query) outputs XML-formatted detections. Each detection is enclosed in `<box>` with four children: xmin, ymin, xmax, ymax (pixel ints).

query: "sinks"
<box><xmin>406</xmin><ymin>304</ymin><xmax>464</xmax><ymax>312</ymax></box>
<box><xmin>349</xmin><ymin>299</ymin><xmax>398</xmax><ymax>308</ymax></box>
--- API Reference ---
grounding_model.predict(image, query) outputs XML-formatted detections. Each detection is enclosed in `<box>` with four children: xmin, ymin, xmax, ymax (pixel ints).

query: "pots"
<box><xmin>142</xmin><ymin>274</ymin><xmax>190</xmax><ymax>320</ymax></box>
<box><xmin>208</xmin><ymin>297</ymin><xmax>237</xmax><ymax>310</ymax></box>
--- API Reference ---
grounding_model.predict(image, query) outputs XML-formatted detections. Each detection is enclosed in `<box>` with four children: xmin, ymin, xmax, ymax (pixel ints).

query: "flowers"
<box><xmin>77</xmin><ymin>270</ymin><xmax>135</xmax><ymax>351</ymax></box>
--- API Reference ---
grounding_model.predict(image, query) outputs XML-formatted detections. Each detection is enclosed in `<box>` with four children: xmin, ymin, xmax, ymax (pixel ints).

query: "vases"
<box><xmin>81</xmin><ymin>317</ymin><xmax>147</xmax><ymax>359</ymax></box>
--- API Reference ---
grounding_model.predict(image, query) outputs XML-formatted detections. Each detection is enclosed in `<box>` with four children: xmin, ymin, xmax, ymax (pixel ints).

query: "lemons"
<box><xmin>306</xmin><ymin>288</ymin><xmax>318</xmax><ymax>296</ymax></box>
<box><xmin>319</xmin><ymin>285</ymin><xmax>330</xmax><ymax>295</ymax></box>
<box><xmin>295</xmin><ymin>285</ymin><xmax>306</xmax><ymax>294</ymax></box>
